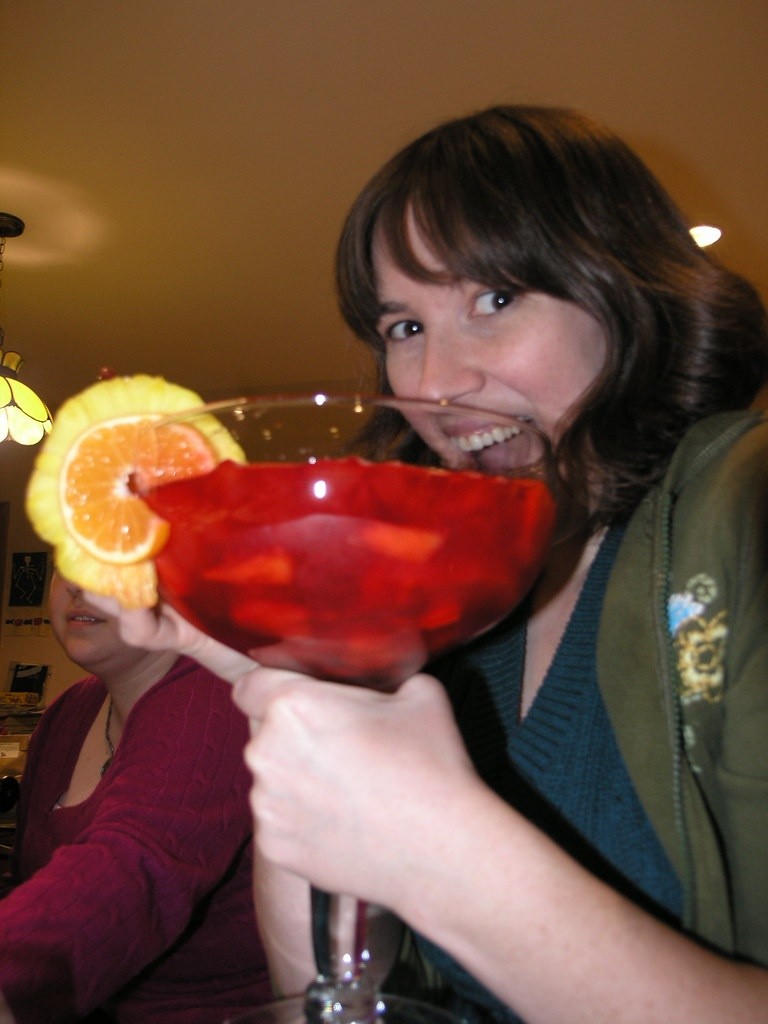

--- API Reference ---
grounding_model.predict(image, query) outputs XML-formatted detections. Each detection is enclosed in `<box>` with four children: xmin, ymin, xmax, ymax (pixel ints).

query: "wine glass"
<box><xmin>129</xmin><ymin>394</ymin><xmax>561</xmax><ymax>1024</ymax></box>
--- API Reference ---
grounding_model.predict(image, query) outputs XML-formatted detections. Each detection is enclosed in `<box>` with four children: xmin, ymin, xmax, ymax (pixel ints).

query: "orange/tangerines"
<box><xmin>56</xmin><ymin>415</ymin><xmax>213</xmax><ymax>562</ymax></box>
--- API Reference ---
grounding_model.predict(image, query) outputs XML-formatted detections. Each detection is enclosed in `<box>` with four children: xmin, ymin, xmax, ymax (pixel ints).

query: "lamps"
<box><xmin>0</xmin><ymin>328</ymin><xmax>54</xmax><ymax>446</ymax></box>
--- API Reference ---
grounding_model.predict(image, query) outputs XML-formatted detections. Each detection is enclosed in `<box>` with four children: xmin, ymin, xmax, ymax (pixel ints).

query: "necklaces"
<box><xmin>100</xmin><ymin>690</ymin><xmax>129</xmax><ymax>777</ymax></box>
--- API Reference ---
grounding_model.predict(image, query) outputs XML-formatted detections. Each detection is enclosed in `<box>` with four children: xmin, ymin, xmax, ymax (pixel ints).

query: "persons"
<box><xmin>77</xmin><ymin>102</ymin><xmax>765</xmax><ymax>1024</ymax></box>
<box><xmin>0</xmin><ymin>554</ymin><xmax>281</xmax><ymax>1024</ymax></box>
